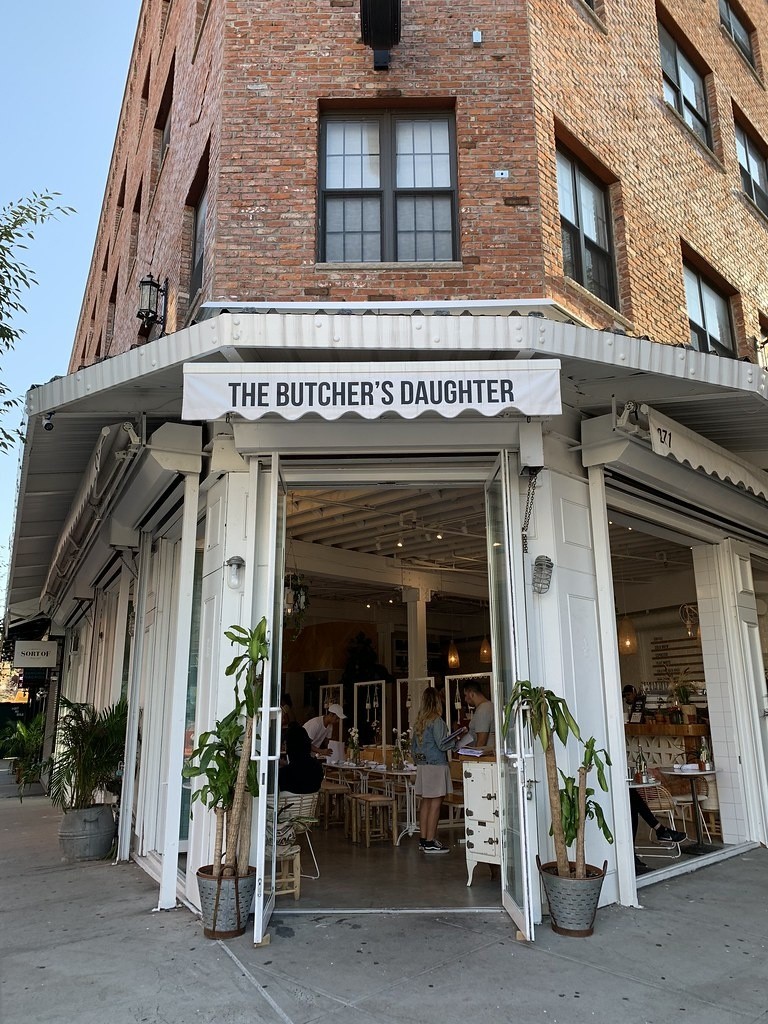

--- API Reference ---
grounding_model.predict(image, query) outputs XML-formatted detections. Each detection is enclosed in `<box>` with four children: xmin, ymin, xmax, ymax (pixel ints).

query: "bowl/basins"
<box><xmin>368</xmin><ymin>761</ymin><xmax>379</xmax><ymax>769</ymax></box>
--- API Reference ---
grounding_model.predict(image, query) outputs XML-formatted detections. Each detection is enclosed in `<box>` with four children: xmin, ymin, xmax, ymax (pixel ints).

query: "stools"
<box><xmin>321</xmin><ymin>743</ymin><xmax>469</xmax><ymax>849</ymax></box>
<box><xmin>265</xmin><ymin>843</ymin><xmax>302</xmax><ymax>902</ymax></box>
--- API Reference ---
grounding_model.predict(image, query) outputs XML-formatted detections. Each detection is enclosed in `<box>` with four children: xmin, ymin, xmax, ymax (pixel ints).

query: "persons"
<box><xmin>464</xmin><ymin>680</ymin><xmax>495</xmax><ymax>748</ymax></box>
<box><xmin>303</xmin><ymin>704</ymin><xmax>347</xmax><ymax>758</ymax></box>
<box><xmin>622</xmin><ymin>684</ymin><xmax>644</xmax><ymax>712</ymax></box>
<box><xmin>278</xmin><ymin>726</ymin><xmax>324</xmax><ymax>794</ymax></box>
<box><xmin>411</xmin><ymin>687</ymin><xmax>465</xmax><ymax>853</ymax></box>
<box><xmin>629</xmin><ymin>788</ymin><xmax>687</xmax><ymax>870</ymax></box>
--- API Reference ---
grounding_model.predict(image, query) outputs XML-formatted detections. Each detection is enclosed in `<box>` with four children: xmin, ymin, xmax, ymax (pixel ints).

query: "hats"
<box><xmin>328</xmin><ymin>704</ymin><xmax>347</xmax><ymax>719</ymax></box>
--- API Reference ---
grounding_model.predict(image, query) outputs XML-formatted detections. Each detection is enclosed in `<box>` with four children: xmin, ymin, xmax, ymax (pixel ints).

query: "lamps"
<box><xmin>479</xmin><ymin>604</ymin><xmax>491</xmax><ymax>664</ymax></box>
<box><xmin>437</xmin><ymin>529</ymin><xmax>444</xmax><ymax>540</ymax></box>
<box><xmin>425</xmin><ymin>529</ymin><xmax>432</xmax><ymax>541</ymax></box>
<box><xmin>461</xmin><ymin>521</ymin><xmax>468</xmax><ymax>534</ymax></box>
<box><xmin>135</xmin><ymin>269</ymin><xmax>171</xmax><ymax>329</ymax></box>
<box><xmin>374</xmin><ymin>538</ymin><xmax>382</xmax><ymax>551</ymax></box>
<box><xmin>447</xmin><ymin>601</ymin><xmax>460</xmax><ymax>669</ymax></box>
<box><xmin>396</xmin><ymin>534</ymin><xmax>405</xmax><ymax>548</ymax></box>
<box><xmin>618</xmin><ymin>555</ymin><xmax>638</xmax><ymax>657</ymax></box>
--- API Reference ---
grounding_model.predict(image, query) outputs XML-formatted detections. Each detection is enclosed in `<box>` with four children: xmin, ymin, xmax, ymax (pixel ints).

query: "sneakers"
<box><xmin>418</xmin><ymin>842</ymin><xmax>426</xmax><ymax>850</ymax></box>
<box><xmin>656</xmin><ymin>827</ymin><xmax>687</xmax><ymax>843</ymax></box>
<box><xmin>424</xmin><ymin>840</ymin><xmax>450</xmax><ymax>854</ymax></box>
<box><xmin>636</xmin><ymin>857</ymin><xmax>648</xmax><ymax>867</ymax></box>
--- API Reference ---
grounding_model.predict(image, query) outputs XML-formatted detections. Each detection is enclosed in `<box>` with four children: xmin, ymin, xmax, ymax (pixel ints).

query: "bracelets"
<box><xmin>456</xmin><ymin>736</ymin><xmax>461</xmax><ymax>740</ymax></box>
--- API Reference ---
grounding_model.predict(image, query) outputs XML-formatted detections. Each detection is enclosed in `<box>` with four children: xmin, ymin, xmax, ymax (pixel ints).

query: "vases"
<box><xmin>394</xmin><ymin>749</ymin><xmax>408</xmax><ymax>769</ymax></box>
<box><xmin>347</xmin><ymin>745</ymin><xmax>360</xmax><ymax>762</ymax></box>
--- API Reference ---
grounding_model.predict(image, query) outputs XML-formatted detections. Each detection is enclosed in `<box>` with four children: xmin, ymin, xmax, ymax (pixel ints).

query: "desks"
<box><xmin>322</xmin><ymin>760</ymin><xmax>375</xmax><ymax>829</ymax></box>
<box><xmin>628</xmin><ymin>779</ymin><xmax>664</xmax><ymax>789</ymax></box>
<box><xmin>660</xmin><ymin>765</ymin><xmax>719</xmax><ymax>848</ymax></box>
<box><xmin>360</xmin><ymin>768</ymin><xmax>420</xmax><ymax>846</ymax></box>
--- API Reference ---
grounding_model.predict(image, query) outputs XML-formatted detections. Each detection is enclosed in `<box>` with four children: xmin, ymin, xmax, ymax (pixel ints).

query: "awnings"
<box><xmin>642</xmin><ymin>404</ymin><xmax>768</xmax><ymax>500</ymax></box>
<box><xmin>38</xmin><ymin>422</ymin><xmax>122</xmax><ymax>613</ymax></box>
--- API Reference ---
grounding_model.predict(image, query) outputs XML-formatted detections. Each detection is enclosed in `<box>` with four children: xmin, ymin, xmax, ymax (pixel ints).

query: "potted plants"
<box><xmin>0</xmin><ymin>710</ymin><xmax>48</xmax><ymax>787</ymax></box>
<box><xmin>182</xmin><ymin>618</ymin><xmax>275</xmax><ymax>939</ymax></box>
<box><xmin>674</xmin><ymin>743</ymin><xmax>712</xmax><ymax>770</ymax></box>
<box><xmin>54</xmin><ymin>697</ymin><xmax>132</xmax><ymax>864</ymax></box>
<box><xmin>489</xmin><ymin>681</ymin><xmax>618</xmax><ymax>936</ymax></box>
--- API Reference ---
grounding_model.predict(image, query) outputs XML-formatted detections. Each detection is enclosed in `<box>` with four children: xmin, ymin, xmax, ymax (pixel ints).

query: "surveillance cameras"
<box><xmin>42</xmin><ymin>419</ymin><xmax>54</xmax><ymax>431</ymax></box>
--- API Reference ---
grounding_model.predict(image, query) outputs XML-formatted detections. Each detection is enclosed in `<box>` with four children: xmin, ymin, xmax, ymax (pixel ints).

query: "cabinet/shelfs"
<box><xmin>459</xmin><ymin>753</ymin><xmax>511</xmax><ymax>888</ymax></box>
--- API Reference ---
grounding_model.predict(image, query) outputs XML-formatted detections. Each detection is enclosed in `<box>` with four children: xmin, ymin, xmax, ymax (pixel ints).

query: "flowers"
<box><xmin>347</xmin><ymin>728</ymin><xmax>363</xmax><ymax>752</ymax></box>
<box><xmin>390</xmin><ymin>727</ymin><xmax>415</xmax><ymax>756</ymax></box>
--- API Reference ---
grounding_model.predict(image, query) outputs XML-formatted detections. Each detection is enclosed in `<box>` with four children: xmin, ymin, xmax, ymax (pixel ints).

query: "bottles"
<box><xmin>636</xmin><ymin>745</ymin><xmax>648</xmax><ymax>775</ymax></box>
<box><xmin>699</xmin><ymin>736</ymin><xmax>711</xmax><ymax>771</ymax></box>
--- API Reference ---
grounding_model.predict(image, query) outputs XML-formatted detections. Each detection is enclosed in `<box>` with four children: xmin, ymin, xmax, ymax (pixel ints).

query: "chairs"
<box><xmin>631</xmin><ymin>767</ymin><xmax>721</xmax><ymax>861</ymax></box>
